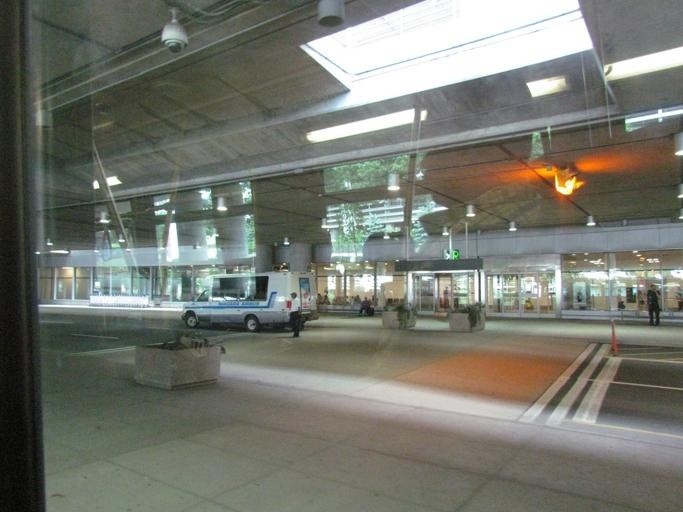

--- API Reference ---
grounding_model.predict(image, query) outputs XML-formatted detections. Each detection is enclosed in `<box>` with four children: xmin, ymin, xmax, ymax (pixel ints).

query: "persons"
<box><xmin>616</xmin><ymin>298</ymin><xmax>625</xmax><ymax>311</ymax></box>
<box><xmin>646</xmin><ymin>284</ymin><xmax>660</xmax><ymax>326</ymax></box>
<box><xmin>288</xmin><ymin>292</ymin><xmax>301</xmax><ymax>338</ymax></box>
<box><xmin>576</xmin><ymin>289</ymin><xmax>582</xmax><ymax>302</ymax></box>
<box><xmin>317</xmin><ymin>293</ymin><xmax>376</xmax><ymax>318</ymax></box>
<box><xmin>442</xmin><ymin>286</ymin><xmax>448</xmax><ymax>307</ymax></box>
<box><xmin>675</xmin><ymin>286</ymin><xmax>683</xmax><ymax>312</ymax></box>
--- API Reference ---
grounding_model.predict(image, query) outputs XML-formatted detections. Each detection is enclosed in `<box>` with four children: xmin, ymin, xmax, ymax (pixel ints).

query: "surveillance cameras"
<box><xmin>161</xmin><ymin>20</ymin><xmax>188</xmax><ymax>54</ymax></box>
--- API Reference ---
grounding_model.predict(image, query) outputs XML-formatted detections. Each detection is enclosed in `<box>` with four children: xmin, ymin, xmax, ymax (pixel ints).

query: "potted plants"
<box><xmin>444</xmin><ymin>302</ymin><xmax>488</xmax><ymax>333</ymax></box>
<box><xmin>127</xmin><ymin>323</ymin><xmax>234</xmax><ymax>393</ymax></box>
<box><xmin>378</xmin><ymin>301</ymin><xmax>418</xmax><ymax>331</ymax></box>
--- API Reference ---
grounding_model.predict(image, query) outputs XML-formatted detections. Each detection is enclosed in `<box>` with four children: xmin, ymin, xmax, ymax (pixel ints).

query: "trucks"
<box><xmin>179</xmin><ymin>271</ymin><xmax>321</xmax><ymax>332</ymax></box>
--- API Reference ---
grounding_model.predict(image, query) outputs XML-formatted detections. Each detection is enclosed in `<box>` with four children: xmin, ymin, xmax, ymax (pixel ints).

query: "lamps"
<box><xmin>441</xmin><ymin>225</ymin><xmax>449</xmax><ymax>235</ymax></box>
<box><xmin>466</xmin><ymin>203</ymin><xmax>476</xmax><ymax>217</ymax></box>
<box><xmin>585</xmin><ymin>214</ymin><xmax>596</xmax><ymax>226</ymax></box>
<box><xmin>383</xmin><ymin>232</ymin><xmax>389</xmax><ymax>239</ymax></box>
<box><xmin>216</xmin><ymin>196</ymin><xmax>228</xmax><ymax>213</ymax></box>
<box><xmin>313</xmin><ymin>1</ymin><xmax>346</xmax><ymax>28</ymax></box>
<box><xmin>35</xmin><ymin>111</ymin><xmax>51</xmax><ymax>130</ymax></box>
<box><xmin>509</xmin><ymin>221</ymin><xmax>517</xmax><ymax>231</ymax></box>
<box><xmin>387</xmin><ymin>173</ymin><xmax>401</xmax><ymax>192</ymax></box>
<box><xmin>670</xmin><ymin>128</ymin><xmax>683</xmax><ymax>223</ymax></box>
<box><xmin>321</xmin><ymin>218</ymin><xmax>327</xmax><ymax>228</ymax></box>
